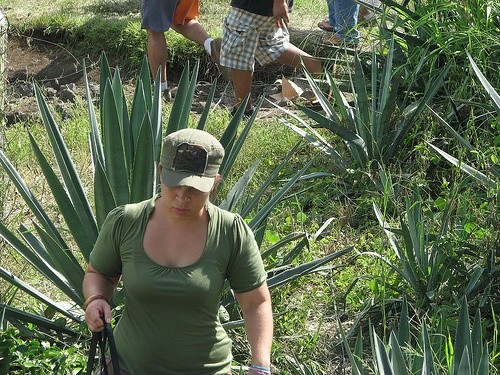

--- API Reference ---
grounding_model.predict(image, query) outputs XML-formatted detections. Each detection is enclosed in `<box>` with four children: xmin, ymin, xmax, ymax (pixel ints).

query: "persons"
<box><xmin>140</xmin><ymin>0</ymin><xmax>223</xmax><ymax>102</ymax></box>
<box><xmin>318</xmin><ymin>0</ymin><xmax>361</xmax><ymax>49</ymax></box>
<box><xmin>219</xmin><ymin>0</ymin><xmax>336</xmax><ymax>120</ymax></box>
<box><xmin>76</xmin><ymin>128</ymin><xmax>276</xmax><ymax>375</ymax></box>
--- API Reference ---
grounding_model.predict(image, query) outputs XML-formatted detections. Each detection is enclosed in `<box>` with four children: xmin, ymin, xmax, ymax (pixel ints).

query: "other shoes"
<box><xmin>318</xmin><ymin>19</ymin><xmax>357</xmax><ymax>44</ymax></box>
<box><xmin>231</xmin><ymin>105</ymin><xmax>254</xmax><ymax>117</ymax></box>
<box><xmin>161</xmin><ymin>89</ymin><xmax>170</xmax><ymax>100</ymax></box>
<box><xmin>210</xmin><ymin>37</ymin><xmax>232</xmax><ymax>83</ymax></box>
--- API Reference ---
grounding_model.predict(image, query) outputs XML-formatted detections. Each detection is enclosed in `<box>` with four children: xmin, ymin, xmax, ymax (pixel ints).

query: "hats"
<box><xmin>157</xmin><ymin>129</ymin><xmax>225</xmax><ymax>191</ymax></box>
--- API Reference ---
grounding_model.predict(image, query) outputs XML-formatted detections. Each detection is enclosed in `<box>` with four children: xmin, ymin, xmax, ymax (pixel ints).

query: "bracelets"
<box><xmin>84</xmin><ymin>294</ymin><xmax>110</xmax><ymax>311</ymax></box>
<box><xmin>249</xmin><ymin>364</ymin><xmax>270</xmax><ymax>375</ymax></box>
